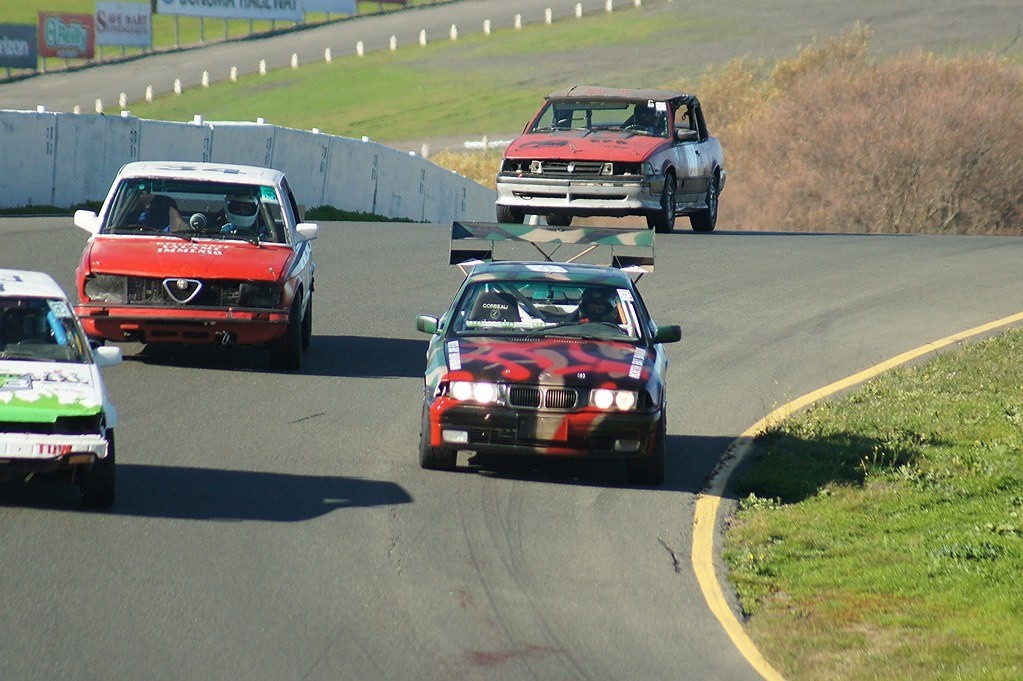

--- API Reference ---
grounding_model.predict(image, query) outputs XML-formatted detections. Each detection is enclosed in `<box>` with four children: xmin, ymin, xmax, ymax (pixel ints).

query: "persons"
<box><xmin>611</xmin><ymin>102</ymin><xmax>661</xmax><ymax>136</ymax></box>
<box><xmin>215</xmin><ymin>189</ymin><xmax>268</xmax><ymax>242</ymax></box>
<box><xmin>578</xmin><ymin>287</ymin><xmax>623</xmax><ymax>326</ymax></box>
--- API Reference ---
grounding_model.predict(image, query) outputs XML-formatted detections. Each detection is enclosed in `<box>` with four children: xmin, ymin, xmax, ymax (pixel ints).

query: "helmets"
<box><xmin>579</xmin><ymin>284</ymin><xmax>617</xmax><ymax>322</ymax></box>
<box><xmin>634</xmin><ymin>104</ymin><xmax>661</xmax><ymax>125</ymax></box>
<box><xmin>224</xmin><ymin>193</ymin><xmax>260</xmax><ymax>227</ymax></box>
<box><xmin>11</xmin><ymin>307</ymin><xmax>50</xmax><ymax>343</ymax></box>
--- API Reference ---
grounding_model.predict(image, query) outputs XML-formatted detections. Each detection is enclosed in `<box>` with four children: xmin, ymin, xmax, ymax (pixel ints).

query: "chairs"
<box><xmin>468</xmin><ymin>291</ymin><xmax>521</xmax><ymax>322</ymax></box>
<box><xmin>119</xmin><ymin>194</ymin><xmax>177</xmax><ymax>233</ymax></box>
<box><xmin>0</xmin><ymin>307</ymin><xmax>58</xmax><ymax>352</ymax></box>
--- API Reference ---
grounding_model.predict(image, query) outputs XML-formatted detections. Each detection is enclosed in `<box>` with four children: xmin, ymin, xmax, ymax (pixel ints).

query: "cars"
<box><xmin>74</xmin><ymin>161</ymin><xmax>319</xmax><ymax>372</ymax></box>
<box><xmin>0</xmin><ymin>268</ymin><xmax>124</xmax><ymax>507</ymax></box>
<box><xmin>495</xmin><ymin>85</ymin><xmax>726</xmax><ymax>234</ymax></box>
<box><xmin>416</xmin><ymin>219</ymin><xmax>681</xmax><ymax>485</ymax></box>
